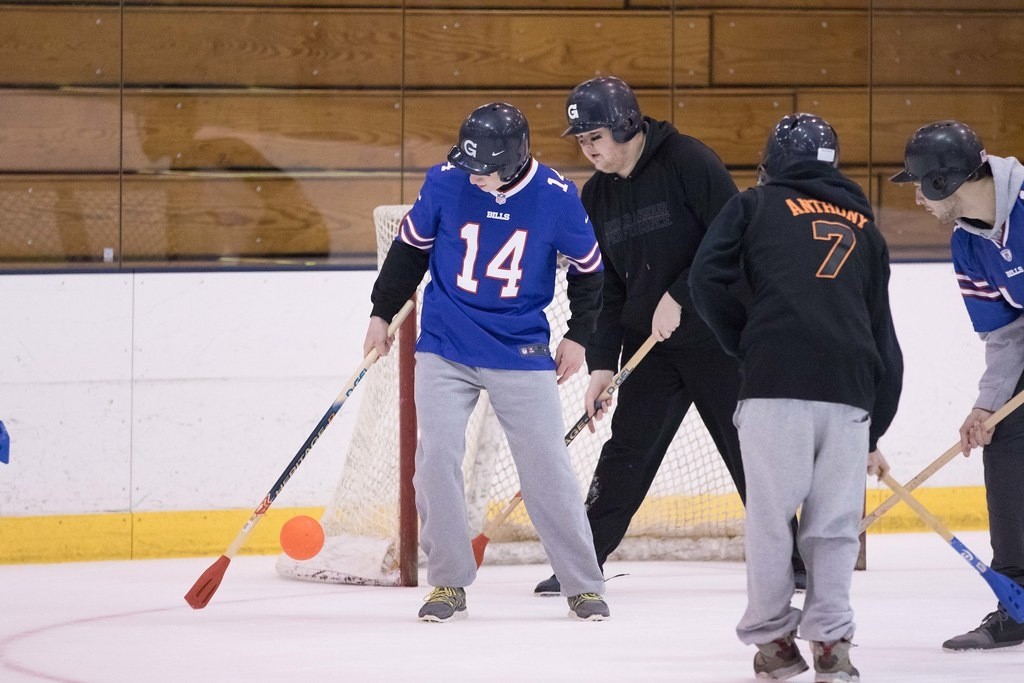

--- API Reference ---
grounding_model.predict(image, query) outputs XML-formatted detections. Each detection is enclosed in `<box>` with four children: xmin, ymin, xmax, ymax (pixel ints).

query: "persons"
<box><xmin>364</xmin><ymin>103</ymin><xmax>611</xmax><ymax>623</ymax></box>
<box><xmin>534</xmin><ymin>76</ymin><xmax>809</xmax><ymax>598</ymax></box>
<box><xmin>687</xmin><ymin>112</ymin><xmax>903</xmax><ymax>683</ymax></box>
<box><xmin>889</xmin><ymin>121</ymin><xmax>1023</xmax><ymax>654</ymax></box>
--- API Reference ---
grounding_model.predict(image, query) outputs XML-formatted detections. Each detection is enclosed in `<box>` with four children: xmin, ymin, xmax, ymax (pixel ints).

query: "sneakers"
<box><xmin>810</xmin><ymin>641</ymin><xmax>859</xmax><ymax>683</ymax></box>
<box><xmin>568</xmin><ymin>594</ymin><xmax>611</xmax><ymax>622</ymax></box>
<box><xmin>417</xmin><ymin>586</ymin><xmax>469</xmax><ymax>622</ymax></box>
<box><xmin>753</xmin><ymin>637</ymin><xmax>809</xmax><ymax>679</ymax></box>
<box><xmin>794</xmin><ymin>569</ymin><xmax>808</xmax><ymax>591</ymax></box>
<box><xmin>942</xmin><ymin>604</ymin><xmax>1024</xmax><ymax>651</ymax></box>
<box><xmin>534</xmin><ymin>573</ymin><xmax>562</xmax><ymax>598</ymax></box>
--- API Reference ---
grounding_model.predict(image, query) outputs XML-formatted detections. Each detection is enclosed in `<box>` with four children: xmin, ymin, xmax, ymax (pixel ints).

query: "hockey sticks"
<box><xmin>876</xmin><ymin>467</ymin><xmax>1024</xmax><ymax>625</ymax></box>
<box><xmin>861</xmin><ymin>388</ymin><xmax>1024</xmax><ymax>531</ymax></box>
<box><xmin>185</xmin><ymin>299</ymin><xmax>418</xmax><ymax>610</ymax></box>
<box><xmin>472</xmin><ymin>331</ymin><xmax>660</xmax><ymax>567</ymax></box>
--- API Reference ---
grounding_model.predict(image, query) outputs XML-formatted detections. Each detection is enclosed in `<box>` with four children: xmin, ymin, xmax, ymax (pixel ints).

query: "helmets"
<box><xmin>770</xmin><ymin>112</ymin><xmax>839</xmax><ymax>168</ymax></box>
<box><xmin>560</xmin><ymin>76</ymin><xmax>643</xmax><ymax>143</ymax></box>
<box><xmin>447</xmin><ymin>103</ymin><xmax>529</xmax><ymax>182</ymax></box>
<box><xmin>891</xmin><ymin>120</ymin><xmax>985</xmax><ymax>201</ymax></box>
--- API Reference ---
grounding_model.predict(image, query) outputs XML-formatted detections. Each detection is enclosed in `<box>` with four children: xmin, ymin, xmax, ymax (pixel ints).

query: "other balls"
<box><xmin>280</xmin><ymin>514</ymin><xmax>328</xmax><ymax>562</ymax></box>
